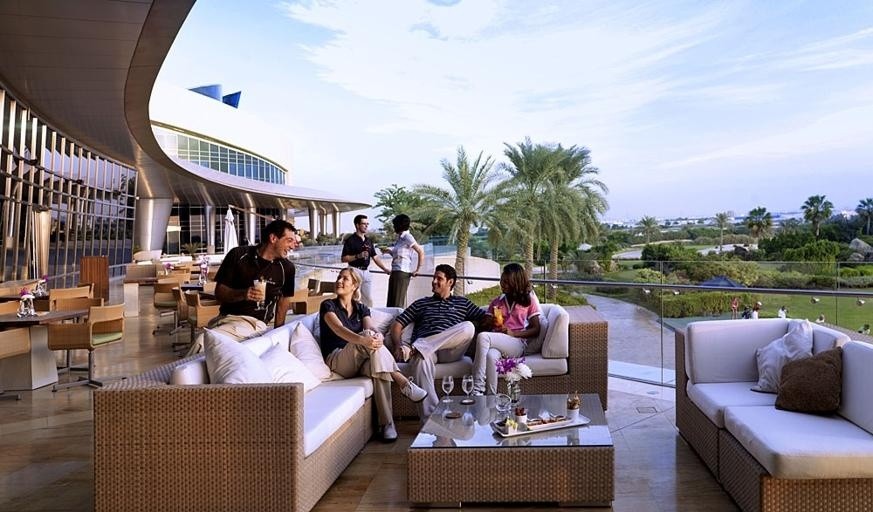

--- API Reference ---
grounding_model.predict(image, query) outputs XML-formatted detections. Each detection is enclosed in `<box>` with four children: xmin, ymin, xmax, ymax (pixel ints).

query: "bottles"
<box><xmin>492</xmin><ymin>305</ymin><xmax>503</xmax><ymax>327</ymax></box>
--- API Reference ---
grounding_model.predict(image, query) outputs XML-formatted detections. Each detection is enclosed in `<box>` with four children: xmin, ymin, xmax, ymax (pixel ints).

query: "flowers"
<box><xmin>494</xmin><ymin>355</ymin><xmax>533</xmax><ymax>385</ymax></box>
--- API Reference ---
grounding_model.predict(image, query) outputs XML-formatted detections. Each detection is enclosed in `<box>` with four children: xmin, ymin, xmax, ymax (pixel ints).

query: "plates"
<box><xmin>488</xmin><ymin>412</ymin><xmax>591</xmax><ymax>437</ymax></box>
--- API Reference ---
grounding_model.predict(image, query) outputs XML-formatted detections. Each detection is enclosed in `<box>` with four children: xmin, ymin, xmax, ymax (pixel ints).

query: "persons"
<box><xmin>319</xmin><ymin>267</ymin><xmax>429</xmax><ymax>441</ymax></box>
<box><xmin>341</xmin><ymin>215</ymin><xmax>391</xmax><ymax>308</ymax></box>
<box><xmin>185</xmin><ymin>219</ymin><xmax>297</xmax><ymax>358</ymax></box>
<box><xmin>379</xmin><ymin>214</ymin><xmax>424</xmax><ymax>308</ymax></box>
<box><xmin>390</xmin><ymin>264</ymin><xmax>488</xmax><ymax>427</ymax></box>
<box><xmin>471</xmin><ymin>263</ymin><xmax>540</xmax><ymax>396</ymax></box>
<box><xmin>729</xmin><ymin>296</ymin><xmax>870</xmax><ymax>336</ymax></box>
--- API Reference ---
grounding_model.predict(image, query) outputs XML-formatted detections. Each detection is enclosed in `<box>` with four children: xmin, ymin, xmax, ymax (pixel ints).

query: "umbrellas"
<box><xmin>699</xmin><ymin>275</ymin><xmax>742</xmax><ymax>311</ymax></box>
<box><xmin>223</xmin><ymin>207</ymin><xmax>238</xmax><ymax>257</ymax></box>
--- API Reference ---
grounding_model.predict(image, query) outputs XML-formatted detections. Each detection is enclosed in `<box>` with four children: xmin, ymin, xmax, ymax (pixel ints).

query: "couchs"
<box><xmin>93</xmin><ymin>306</ymin><xmax>607</xmax><ymax>512</ymax></box>
<box><xmin>675</xmin><ymin>318</ymin><xmax>873</xmax><ymax>512</ymax></box>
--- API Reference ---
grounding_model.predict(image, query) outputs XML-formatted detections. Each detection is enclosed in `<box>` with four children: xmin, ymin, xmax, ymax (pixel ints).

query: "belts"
<box><xmin>358</xmin><ymin>267</ymin><xmax>367</xmax><ymax>269</ymax></box>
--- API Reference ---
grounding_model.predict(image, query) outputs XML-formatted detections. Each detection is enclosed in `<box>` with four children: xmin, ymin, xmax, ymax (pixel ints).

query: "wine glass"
<box><xmin>460</xmin><ymin>375</ymin><xmax>474</xmax><ymax>403</ymax></box>
<box><xmin>460</xmin><ymin>404</ymin><xmax>474</xmax><ymax>430</ymax></box>
<box><xmin>493</xmin><ymin>394</ymin><xmax>511</xmax><ymax>422</ymax></box>
<box><xmin>252</xmin><ymin>280</ymin><xmax>268</xmax><ymax>313</ymax></box>
<box><xmin>441</xmin><ymin>375</ymin><xmax>454</xmax><ymax>403</ymax></box>
<box><xmin>441</xmin><ymin>402</ymin><xmax>453</xmax><ymax>424</ymax></box>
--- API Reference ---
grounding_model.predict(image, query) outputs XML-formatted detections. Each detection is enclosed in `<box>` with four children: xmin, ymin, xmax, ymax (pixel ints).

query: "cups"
<box><xmin>363</xmin><ymin>244</ymin><xmax>368</xmax><ymax>252</ymax></box>
<box><xmin>567</xmin><ymin>408</ymin><xmax>580</xmax><ymax>419</ymax></box>
<box><xmin>514</xmin><ymin>415</ymin><xmax>530</xmax><ymax>429</ymax></box>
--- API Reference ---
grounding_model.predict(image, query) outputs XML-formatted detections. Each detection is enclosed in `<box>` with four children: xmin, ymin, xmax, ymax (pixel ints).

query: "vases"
<box><xmin>507</xmin><ymin>384</ymin><xmax>521</xmax><ymax>403</ymax></box>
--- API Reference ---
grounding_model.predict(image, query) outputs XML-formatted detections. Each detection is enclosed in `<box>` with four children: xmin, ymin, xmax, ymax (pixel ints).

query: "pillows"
<box><xmin>368</xmin><ymin>309</ymin><xmax>392</xmax><ymax>333</ymax></box>
<box><xmin>386</xmin><ymin>309</ymin><xmax>413</xmax><ymax>347</ymax></box>
<box><xmin>774</xmin><ymin>346</ymin><xmax>843</xmax><ymax>418</ymax></box>
<box><xmin>521</xmin><ymin>291</ymin><xmax>549</xmax><ymax>354</ymax></box>
<box><xmin>751</xmin><ymin>318</ymin><xmax>814</xmax><ymax>392</ymax></box>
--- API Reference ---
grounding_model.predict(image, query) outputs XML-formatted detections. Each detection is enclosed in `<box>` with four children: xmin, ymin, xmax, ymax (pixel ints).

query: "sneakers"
<box><xmin>377</xmin><ymin>420</ymin><xmax>398</xmax><ymax>440</ymax></box>
<box><xmin>392</xmin><ymin>345</ymin><xmax>415</xmax><ymax>363</ymax></box>
<box><xmin>399</xmin><ymin>376</ymin><xmax>427</xmax><ymax>402</ymax></box>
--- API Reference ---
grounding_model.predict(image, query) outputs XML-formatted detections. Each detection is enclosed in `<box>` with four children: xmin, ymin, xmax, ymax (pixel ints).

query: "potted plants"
<box><xmin>183</xmin><ymin>242</ymin><xmax>201</xmax><ymax>259</ymax></box>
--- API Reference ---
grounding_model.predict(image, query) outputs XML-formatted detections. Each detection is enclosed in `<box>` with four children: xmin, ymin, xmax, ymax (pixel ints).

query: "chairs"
<box><xmin>151</xmin><ymin>263</ymin><xmax>336</xmax><ymax>352</ymax></box>
<box><xmin>0</xmin><ymin>279</ymin><xmax>123</xmax><ymax>402</ymax></box>
